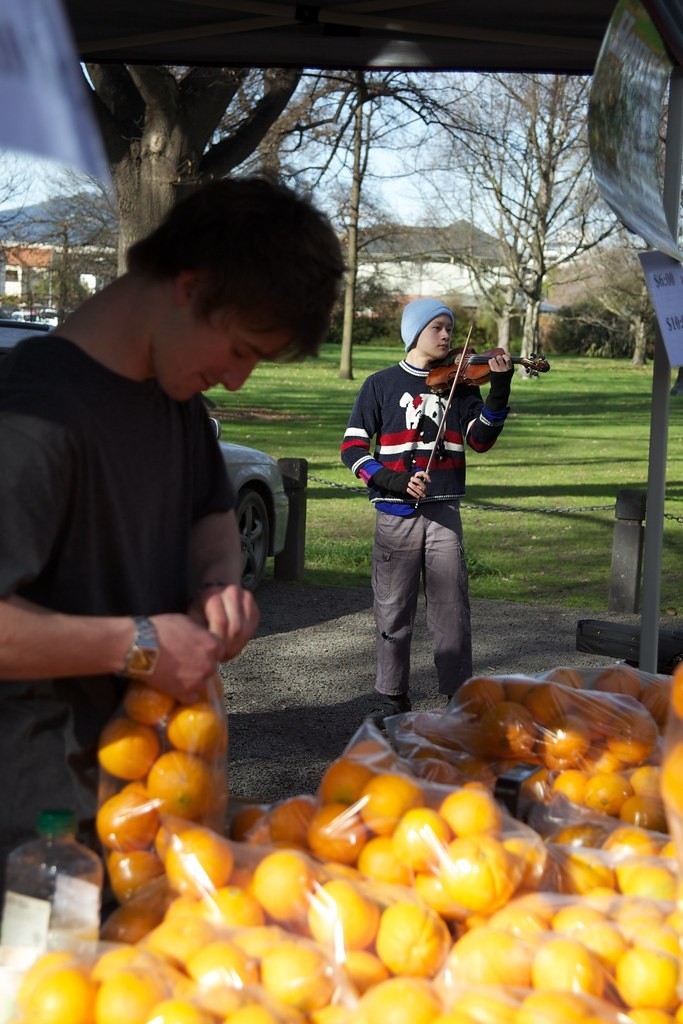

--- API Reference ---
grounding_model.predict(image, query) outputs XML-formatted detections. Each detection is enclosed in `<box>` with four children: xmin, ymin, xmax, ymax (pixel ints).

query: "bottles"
<box><xmin>1</xmin><ymin>810</ymin><xmax>105</xmax><ymax>982</ymax></box>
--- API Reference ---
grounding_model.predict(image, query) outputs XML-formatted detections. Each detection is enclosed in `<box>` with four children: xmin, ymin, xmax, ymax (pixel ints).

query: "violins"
<box><xmin>424</xmin><ymin>346</ymin><xmax>551</xmax><ymax>391</ymax></box>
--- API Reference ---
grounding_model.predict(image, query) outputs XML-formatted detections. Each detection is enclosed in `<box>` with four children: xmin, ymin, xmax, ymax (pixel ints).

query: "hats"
<box><xmin>401</xmin><ymin>299</ymin><xmax>454</xmax><ymax>352</ymax></box>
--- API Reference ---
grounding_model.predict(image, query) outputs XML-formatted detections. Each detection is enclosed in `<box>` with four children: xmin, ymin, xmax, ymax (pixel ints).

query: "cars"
<box><xmin>1</xmin><ymin>321</ymin><xmax>291</xmax><ymax>599</ymax></box>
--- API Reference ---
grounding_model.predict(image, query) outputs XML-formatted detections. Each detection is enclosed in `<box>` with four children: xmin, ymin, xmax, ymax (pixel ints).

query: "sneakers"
<box><xmin>363</xmin><ymin>697</ymin><xmax>412</xmax><ymax>729</ymax></box>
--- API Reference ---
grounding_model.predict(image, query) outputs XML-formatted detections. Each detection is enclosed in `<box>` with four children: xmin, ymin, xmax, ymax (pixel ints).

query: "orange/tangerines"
<box><xmin>5</xmin><ymin>664</ymin><xmax>683</xmax><ymax>1024</ymax></box>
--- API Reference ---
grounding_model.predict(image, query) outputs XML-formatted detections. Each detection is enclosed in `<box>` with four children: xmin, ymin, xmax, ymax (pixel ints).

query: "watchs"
<box><xmin>113</xmin><ymin>616</ymin><xmax>157</xmax><ymax>683</ymax></box>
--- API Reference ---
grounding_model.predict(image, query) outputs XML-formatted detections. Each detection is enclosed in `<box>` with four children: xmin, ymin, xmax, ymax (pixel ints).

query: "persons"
<box><xmin>340</xmin><ymin>299</ymin><xmax>515</xmax><ymax>727</ymax></box>
<box><xmin>0</xmin><ymin>177</ymin><xmax>343</xmax><ymax>913</ymax></box>
